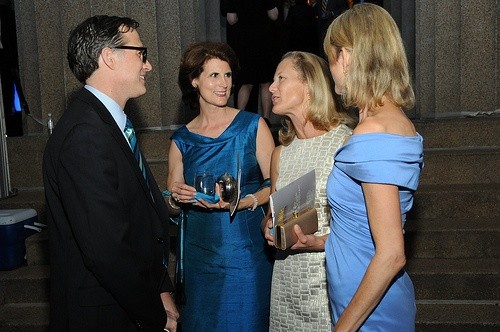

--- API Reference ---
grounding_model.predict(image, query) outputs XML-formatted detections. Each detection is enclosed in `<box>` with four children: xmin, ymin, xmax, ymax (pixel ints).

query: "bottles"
<box><xmin>47</xmin><ymin>113</ymin><xmax>55</xmax><ymax>135</ymax></box>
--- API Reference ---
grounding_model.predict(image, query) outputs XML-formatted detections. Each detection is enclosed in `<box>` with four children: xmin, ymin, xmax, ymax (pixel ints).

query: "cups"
<box><xmin>193</xmin><ymin>170</ymin><xmax>214</xmax><ymax>197</ymax></box>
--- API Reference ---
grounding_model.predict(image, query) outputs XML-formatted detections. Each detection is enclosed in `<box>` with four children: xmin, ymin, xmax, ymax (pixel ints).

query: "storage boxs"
<box><xmin>0</xmin><ymin>208</ymin><xmax>39</xmax><ymax>271</ymax></box>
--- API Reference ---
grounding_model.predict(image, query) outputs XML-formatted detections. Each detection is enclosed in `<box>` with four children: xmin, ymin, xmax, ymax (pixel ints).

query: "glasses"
<box><xmin>110</xmin><ymin>44</ymin><xmax>148</xmax><ymax>64</ymax></box>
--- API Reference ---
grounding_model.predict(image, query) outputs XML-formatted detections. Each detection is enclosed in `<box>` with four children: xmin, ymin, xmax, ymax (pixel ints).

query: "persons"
<box><xmin>1</xmin><ymin>63</ymin><xmax>31</xmax><ymax>138</ymax></box>
<box><xmin>225</xmin><ymin>0</ymin><xmax>383</xmax><ymax>127</ymax></box>
<box><xmin>260</xmin><ymin>50</ymin><xmax>354</xmax><ymax>331</ymax></box>
<box><xmin>165</xmin><ymin>42</ymin><xmax>275</xmax><ymax>331</ymax></box>
<box><xmin>42</xmin><ymin>16</ymin><xmax>180</xmax><ymax>332</ymax></box>
<box><xmin>323</xmin><ymin>3</ymin><xmax>426</xmax><ymax>331</ymax></box>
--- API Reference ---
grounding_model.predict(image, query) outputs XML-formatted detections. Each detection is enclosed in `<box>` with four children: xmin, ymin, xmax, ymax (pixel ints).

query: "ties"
<box><xmin>124</xmin><ymin>117</ymin><xmax>149</xmax><ymax>187</ymax></box>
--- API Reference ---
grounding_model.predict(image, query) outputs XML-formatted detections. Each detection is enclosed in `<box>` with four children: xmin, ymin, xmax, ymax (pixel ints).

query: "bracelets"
<box><xmin>244</xmin><ymin>194</ymin><xmax>257</xmax><ymax>211</ymax></box>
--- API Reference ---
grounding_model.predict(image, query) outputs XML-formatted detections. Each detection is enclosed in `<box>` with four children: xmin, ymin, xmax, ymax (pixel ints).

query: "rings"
<box><xmin>177</xmin><ymin>196</ymin><xmax>180</xmax><ymax>201</ymax></box>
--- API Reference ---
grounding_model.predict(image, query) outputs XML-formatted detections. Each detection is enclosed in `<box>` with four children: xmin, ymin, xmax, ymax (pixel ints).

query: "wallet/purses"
<box><xmin>272</xmin><ymin>207</ymin><xmax>318</xmax><ymax>250</ymax></box>
